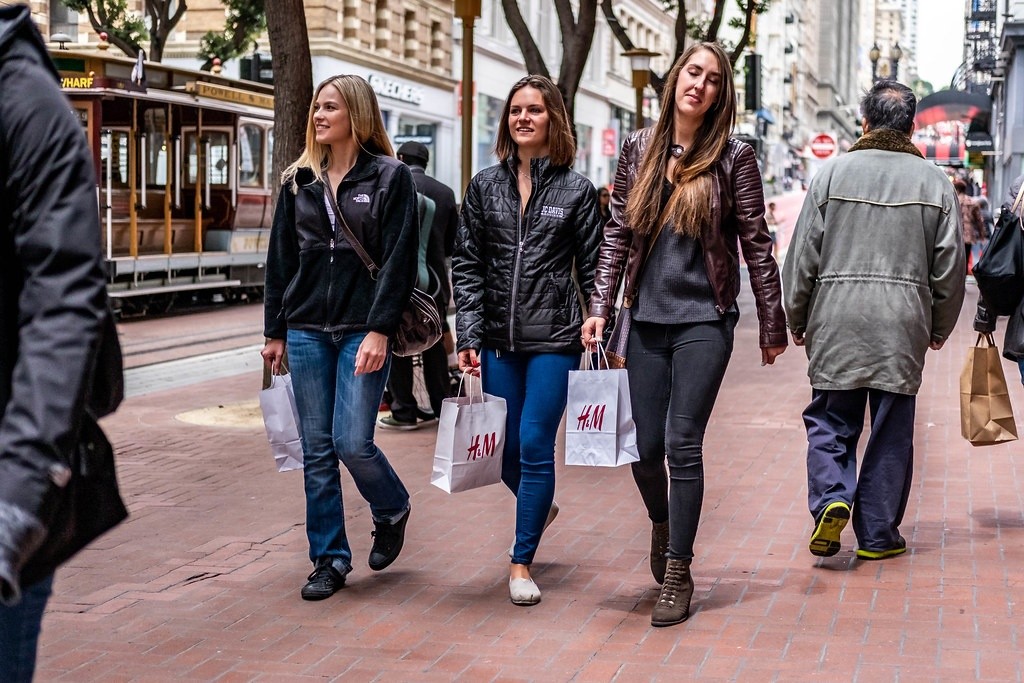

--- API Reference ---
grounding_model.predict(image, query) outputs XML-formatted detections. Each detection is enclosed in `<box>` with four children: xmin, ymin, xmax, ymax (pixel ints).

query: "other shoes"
<box><xmin>378</xmin><ymin>416</ymin><xmax>418</xmax><ymax>430</ymax></box>
<box><xmin>379</xmin><ymin>403</ymin><xmax>392</xmax><ymax>412</ymax></box>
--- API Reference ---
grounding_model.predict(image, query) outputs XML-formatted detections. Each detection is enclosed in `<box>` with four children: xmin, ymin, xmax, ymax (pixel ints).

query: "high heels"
<box><xmin>651</xmin><ymin>560</ymin><xmax>694</xmax><ymax>625</ymax></box>
<box><xmin>651</xmin><ymin>523</ymin><xmax>669</xmax><ymax>585</ymax></box>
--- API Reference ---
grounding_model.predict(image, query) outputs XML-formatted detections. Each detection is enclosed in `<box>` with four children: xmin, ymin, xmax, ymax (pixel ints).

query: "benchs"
<box><xmin>100</xmin><ymin>188</ymin><xmax>212</xmax><ymax>253</ymax></box>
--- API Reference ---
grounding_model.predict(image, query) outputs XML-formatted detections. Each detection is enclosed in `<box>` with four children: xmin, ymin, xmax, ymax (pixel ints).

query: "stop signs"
<box><xmin>810</xmin><ymin>133</ymin><xmax>837</xmax><ymax>160</ymax></box>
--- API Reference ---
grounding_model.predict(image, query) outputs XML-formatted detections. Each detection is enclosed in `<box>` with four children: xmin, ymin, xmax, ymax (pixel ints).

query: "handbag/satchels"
<box><xmin>594</xmin><ymin>287</ymin><xmax>631</xmax><ymax>371</ymax></box>
<box><xmin>971</xmin><ymin>180</ymin><xmax>1024</xmax><ymax>315</ymax></box>
<box><xmin>961</xmin><ymin>332</ymin><xmax>1020</xmax><ymax>447</ymax></box>
<box><xmin>564</xmin><ymin>342</ymin><xmax>641</xmax><ymax>468</ymax></box>
<box><xmin>430</xmin><ymin>367</ymin><xmax>507</xmax><ymax>495</ymax></box>
<box><xmin>388</xmin><ymin>287</ymin><xmax>442</xmax><ymax>357</ymax></box>
<box><xmin>259</xmin><ymin>361</ymin><xmax>305</xmax><ymax>472</ymax></box>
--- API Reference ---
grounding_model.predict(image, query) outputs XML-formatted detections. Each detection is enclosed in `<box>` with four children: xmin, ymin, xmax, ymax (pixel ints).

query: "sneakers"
<box><xmin>302</xmin><ymin>566</ymin><xmax>345</xmax><ymax>599</ymax></box>
<box><xmin>369</xmin><ymin>501</ymin><xmax>411</xmax><ymax>571</ymax></box>
<box><xmin>510</xmin><ymin>569</ymin><xmax>542</xmax><ymax>604</ymax></box>
<box><xmin>809</xmin><ymin>501</ymin><xmax>850</xmax><ymax>557</ymax></box>
<box><xmin>509</xmin><ymin>499</ymin><xmax>559</xmax><ymax>557</ymax></box>
<box><xmin>856</xmin><ymin>536</ymin><xmax>906</xmax><ymax>559</ymax></box>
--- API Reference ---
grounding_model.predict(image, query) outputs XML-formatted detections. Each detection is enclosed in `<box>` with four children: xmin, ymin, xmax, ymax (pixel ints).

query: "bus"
<box><xmin>45</xmin><ymin>32</ymin><xmax>277</xmax><ymax>323</ymax></box>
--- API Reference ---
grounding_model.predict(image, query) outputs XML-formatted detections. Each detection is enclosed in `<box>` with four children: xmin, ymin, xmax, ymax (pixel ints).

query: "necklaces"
<box><xmin>517</xmin><ymin>168</ymin><xmax>531</xmax><ymax>180</ymax></box>
<box><xmin>670</xmin><ymin>145</ymin><xmax>685</xmax><ymax>158</ymax></box>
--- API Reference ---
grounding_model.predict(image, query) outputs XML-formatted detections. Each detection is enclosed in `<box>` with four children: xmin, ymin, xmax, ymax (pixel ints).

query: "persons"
<box><xmin>0</xmin><ymin>2</ymin><xmax>130</xmax><ymax>683</ymax></box>
<box><xmin>597</xmin><ymin>187</ymin><xmax>612</xmax><ymax>223</ymax></box>
<box><xmin>766</xmin><ymin>203</ymin><xmax>788</xmax><ymax>263</ymax></box>
<box><xmin>952</xmin><ymin>176</ymin><xmax>997</xmax><ymax>282</ymax></box>
<box><xmin>378</xmin><ymin>139</ymin><xmax>458</xmax><ymax>430</ymax></box>
<box><xmin>971</xmin><ymin>174</ymin><xmax>1024</xmax><ymax>387</ymax></box>
<box><xmin>452</xmin><ymin>74</ymin><xmax>614</xmax><ymax>604</ymax></box>
<box><xmin>259</xmin><ymin>73</ymin><xmax>420</xmax><ymax>598</ymax></box>
<box><xmin>581</xmin><ymin>42</ymin><xmax>788</xmax><ymax>627</ymax></box>
<box><xmin>782</xmin><ymin>78</ymin><xmax>965</xmax><ymax>560</ymax></box>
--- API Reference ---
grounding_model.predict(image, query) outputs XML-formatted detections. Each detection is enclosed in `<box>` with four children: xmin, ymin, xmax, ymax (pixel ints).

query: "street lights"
<box><xmin>621</xmin><ymin>46</ymin><xmax>663</xmax><ymax>130</ymax></box>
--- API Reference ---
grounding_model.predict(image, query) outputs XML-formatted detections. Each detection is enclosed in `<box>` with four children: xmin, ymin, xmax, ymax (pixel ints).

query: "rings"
<box><xmin>580</xmin><ymin>336</ymin><xmax>585</xmax><ymax>339</ymax></box>
